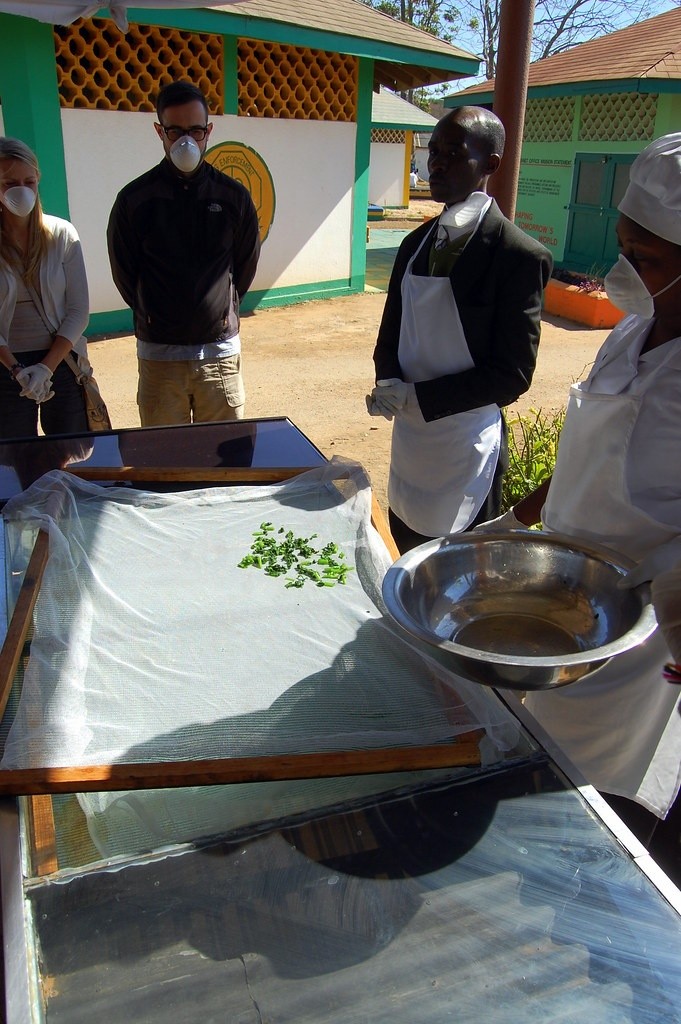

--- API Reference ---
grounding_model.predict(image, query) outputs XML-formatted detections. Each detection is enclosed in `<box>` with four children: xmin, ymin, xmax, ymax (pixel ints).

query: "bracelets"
<box><xmin>8</xmin><ymin>363</ymin><xmax>26</xmax><ymax>380</ymax></box>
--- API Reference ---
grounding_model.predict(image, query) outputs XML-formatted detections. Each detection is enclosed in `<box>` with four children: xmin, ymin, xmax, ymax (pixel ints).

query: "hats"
<box><xmin>617</xmin><ymin>132</ymin><xmax>681</xmax><ymax>246</ymax></box>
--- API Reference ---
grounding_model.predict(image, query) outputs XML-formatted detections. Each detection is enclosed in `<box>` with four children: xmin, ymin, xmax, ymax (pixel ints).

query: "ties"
<box><xmin>434</xmin><ymin>226</ymin><xmax>448</xmax><ymax>253</ymax></box>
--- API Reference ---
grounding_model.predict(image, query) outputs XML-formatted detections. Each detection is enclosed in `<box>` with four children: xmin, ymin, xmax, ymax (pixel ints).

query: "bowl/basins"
<box><xmin>382</xmin><ymin>530</ymin><xmax>658</xmax><ymax>691</ymax></box>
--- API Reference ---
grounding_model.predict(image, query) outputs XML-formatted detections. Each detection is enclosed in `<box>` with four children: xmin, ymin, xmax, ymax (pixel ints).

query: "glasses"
<box><xmin>161</xmin><ymin>122</ymin><xmax>208</xmax><ymax>141</ymax></box>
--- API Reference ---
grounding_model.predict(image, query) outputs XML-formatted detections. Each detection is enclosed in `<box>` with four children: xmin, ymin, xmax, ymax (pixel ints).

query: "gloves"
<box><xmin>366</xmin><ymin>378</ymin><xmax>416</xmax><ymax>421</ymax></box>
<box><xmin>616</xmin><ymin>535</ymin><xmax>681</xmax><ymax>591</ymax></box>
<box><xmin>471</xmin><ymin>505</ymin><xmax>530</xmax><ymax>530</ymax></box>
<box><xmin>15</xmin><ymin>363</ymin><xmax>55</xmax><ymax>404</ymax></box>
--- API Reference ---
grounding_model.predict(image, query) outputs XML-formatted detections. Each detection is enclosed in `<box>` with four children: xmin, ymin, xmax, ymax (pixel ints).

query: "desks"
<box><xmin>0</xmin><ymin>416</ymin><xmax>680</xmax><ymax>1023</ymax></box>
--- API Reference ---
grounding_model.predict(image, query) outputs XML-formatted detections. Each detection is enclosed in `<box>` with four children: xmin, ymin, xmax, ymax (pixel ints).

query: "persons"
<box><xmin>365</xmin><ymin>105</ymin><xmax>554</xmax><ymax>557</ymax></box>
<box><xmin>0</xmin><ymin>136</ymin><xmax>89</xmax><ymax>436</ymax></box>
<box><xmin>414</xmin><ymin>169</ymin><xmax>424</xmax><ymax>182</ymax></box>
<box><xmin>409</xmin><ymin>170</ymin><xmax>418</xmax><ymax>188</ymax></box>
<box><xmin>472</xmin><ymin>131</ymin><xmax>681</xmax><ymax>850</ymax></box>
<box><xmin>106</xmin><ymin>80</ymin><xmax>261</xmax><ymax>428</ymax></box>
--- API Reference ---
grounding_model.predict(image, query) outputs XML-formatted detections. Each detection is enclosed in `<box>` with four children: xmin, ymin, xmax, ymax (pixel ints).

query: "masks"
<box><xmin>603</xmin><ymin>253</ymin><xmax>681</xmax><ymax>320</ymax></box>
<box><xmin>3</xmin><ymin>186</ymin><xmax>37</xmax><ymax>218</ymax></box>
<box><xmin>169</xmin><ymin>136</ymin><xmax>201</xmax><ymax>172</ymax></box>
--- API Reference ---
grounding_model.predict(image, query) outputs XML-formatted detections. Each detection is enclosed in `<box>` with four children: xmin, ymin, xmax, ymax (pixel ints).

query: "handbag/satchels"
<box><xmin>76</xmin><ymin>366</ymin><xmax>112</xmax><ymax>431</ymax></box>
